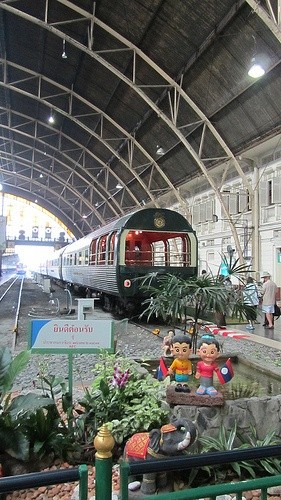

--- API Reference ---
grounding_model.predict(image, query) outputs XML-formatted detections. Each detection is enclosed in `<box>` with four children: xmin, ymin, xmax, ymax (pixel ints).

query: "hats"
<box><xmin>261</xmin><ymin>271</ymin><xmax>271</xmax><ymax>277</ymax></box>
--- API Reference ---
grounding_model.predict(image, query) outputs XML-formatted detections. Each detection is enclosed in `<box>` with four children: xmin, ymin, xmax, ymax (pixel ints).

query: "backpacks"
<box><xmin>272</xmin><ymin>305</ymin><xmax>281</xmax><ymax>321</ymax></box>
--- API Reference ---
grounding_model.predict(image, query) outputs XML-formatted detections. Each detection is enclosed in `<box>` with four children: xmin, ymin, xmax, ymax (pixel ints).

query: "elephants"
<box><xmin>119</xmin><ymin>414</ymin><xmax>200</xmax><ymax>495</ymax></box>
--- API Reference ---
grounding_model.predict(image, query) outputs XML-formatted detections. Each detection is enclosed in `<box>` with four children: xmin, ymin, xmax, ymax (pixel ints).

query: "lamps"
<box><xmin>156</xmin><ymin>146</ymin><xmax>165</xmax><ymax>155</ymax></box>
<box><xmin>116</xmin><ymin>182</ymin><xmax>123</xmax><ymax>189</ymax></box>
<box><xmin>62</xmin><ymin>40</ymin><xmax>67</xmax><ymax>58</ymax></box>
<box><xmin>213</xmin><ymin>215</ymin><xmax>245</xmax><ymax>222</ymax></box>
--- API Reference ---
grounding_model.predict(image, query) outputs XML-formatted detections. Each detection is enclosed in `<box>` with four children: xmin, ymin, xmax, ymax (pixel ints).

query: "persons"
<box><xmin>170</xmin><ymin>335</ymin><xmax>194</xmax><ymax>392</ymax></box>
<box><xmin>164</xmin><ymin>329</ymin><xmax>176</xmax><ymax>358</ymax></box>
<box><xmin>259</xmin><ymin>271</ymin><xmax>278</xmax><ymax>328</ymax></box>
<box><xmin>222</xmin><ymin>276</ymin><xmax>233</xmax><ymax>285</ymax></box>
<box><xmin>201</xmin><ymin>270</ymin><xmax>207</xmax><ymax>279</ymax></box>
<box><xmin>245</xmin><ymin>277</ymin><xmax>259</xmax><ymax>330</ymax></box>
<box><xmin>194</xmin><ymin>333</ymin><xmax>221</xmax><ymax>396</ymax></box>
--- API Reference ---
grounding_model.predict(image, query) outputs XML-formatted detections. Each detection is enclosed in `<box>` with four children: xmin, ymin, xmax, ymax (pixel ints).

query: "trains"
<box><xmin>27</xmin><ymin>207</ymin><xmax>199</xmax><ymax>320</ymax></box>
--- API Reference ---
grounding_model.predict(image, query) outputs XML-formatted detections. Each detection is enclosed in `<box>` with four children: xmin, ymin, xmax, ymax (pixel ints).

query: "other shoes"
<box><xmin>246</xmin><ymin>326</ymin><xmax>255</xmax><ymax>330</ymax></box>
<box><xmin>254</xmin><ymin>320</ymin><xmax>260</xmax><ymax>324</ymax></box>
<box><xmin>266</xmin><ymin>325</ymin><xmax>274</xmax><ymax>329</ymax></box>
<box><xmin>263</xmin><ymin>324</ymin><xmax>266</xmax><ymax>326</ymax></box>
